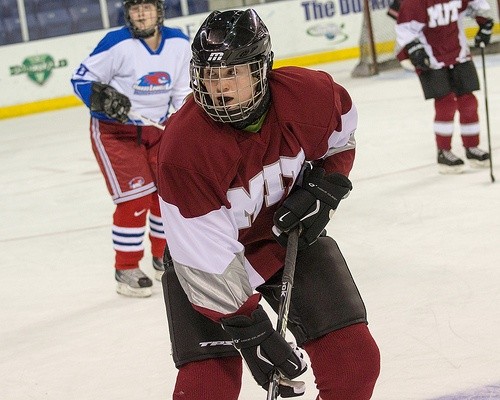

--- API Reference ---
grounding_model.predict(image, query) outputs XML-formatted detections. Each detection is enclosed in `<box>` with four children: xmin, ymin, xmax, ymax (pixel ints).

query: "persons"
<box><xmin>386</xmin><ymin>0</ymin><xmax>495</xmax><ymax>174</ymax></box>
<box><xmin>71</xmin><ymin>0</ymin><xmax>195</xmax><ymax>298</ymax></box>
<box><xmin>155</xmin><ymin>11</ymin><xmax>382</xmax><ymax>400</ymax></box>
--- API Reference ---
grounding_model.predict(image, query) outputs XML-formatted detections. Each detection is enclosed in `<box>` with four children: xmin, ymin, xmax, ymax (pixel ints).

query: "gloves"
<box><xmin>271</xmin><ymin>165</ymin><xmax>353</xmax><ymax>251</ymax></box>
<box><xmin>88</xmin><ymin>81</ymin><xmax>132</xmax><ymax>123</ymax></box>
<box><xmin>474</xmin><ymin>18</ymin><xmax>494</xmax><ymax>49</ymax></box>
<box><xmin>404</xmin><ymin>40</ymin><xmax>430</xmax><ymax>72</ymax></box>
<box><xmin>220</xmin><ymin>303</ymin><xmax>309</xmax><ymax>398</ymax></box>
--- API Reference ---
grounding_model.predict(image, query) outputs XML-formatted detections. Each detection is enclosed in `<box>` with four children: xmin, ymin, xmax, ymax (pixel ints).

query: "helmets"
<box><xmin>190</xmin><ymin>8</ymin><xmax>272</xmax><ymax>68</ymax></box>
<box><xmin>123</xmin><ymin>0</ymin><xmax>164</xmax><ymax>38</ymax></box>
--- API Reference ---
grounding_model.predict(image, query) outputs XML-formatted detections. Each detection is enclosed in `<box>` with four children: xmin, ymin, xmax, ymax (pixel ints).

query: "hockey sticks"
<box><xmin>480</xmin><ymin>41</ymin><xmax>497</xmax><ymax>184</ymax></box>
<box><xmin>264</xmin><ymin>160</ymin><xmax>311</xmax><ymax>400</ymax></box>
<box><xmin>91</xmin><ymin>84</ymin><xmax>165</xmax><ymax>131</ymax></box>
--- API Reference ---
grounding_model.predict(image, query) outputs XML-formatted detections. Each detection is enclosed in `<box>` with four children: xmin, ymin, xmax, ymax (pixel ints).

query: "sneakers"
<box><xmin>114</xmin><ymin>267</ymin><xmax>153</xmax><ymax>297</ymax></box>
<box><xmin>465</xmin><ymin>146</ymin><xmax>490</xmax><ymax>166</ymax></box>
<box><xmin>437</xmin><ymin>150</ymin><xmax>464</xmax><ymax>174</ymax></box>
<box><xmin>152</xmin><ymin>257</ymin><xmax>165</xmax><ymax>281</ymax></box>
